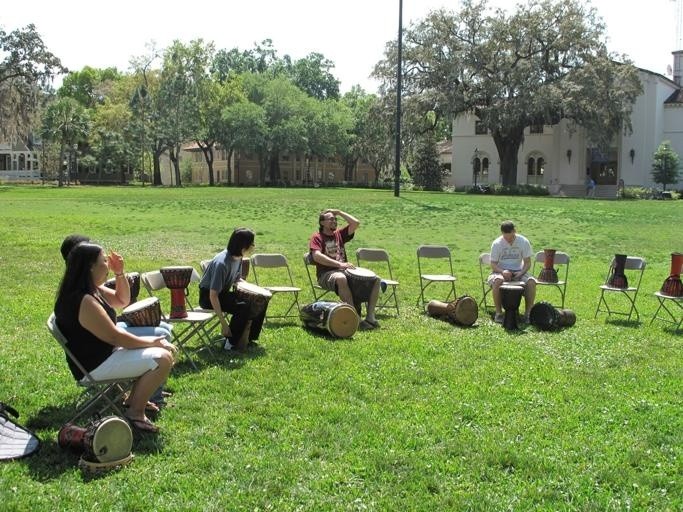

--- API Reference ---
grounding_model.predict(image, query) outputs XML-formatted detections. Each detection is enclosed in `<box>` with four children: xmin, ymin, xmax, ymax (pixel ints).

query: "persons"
<box><xmin>56</xmin><ymin>241</ymin><xmax>178</xmax><ymax>432</ymax></box>
<box><xmin>584</xmin><ymin>176</ymin><xmax>596</xmax><ymax>197</ymax></box>
<box><xmin>61</xmin><ymin>235</ymin><xmax>174</xmax><ymax>408</ymax></box>
<box><xmin>198</xmin><ymin>227</ymin><xmax>268</xmax><ymax>349</ymax></box>
<box><xmin>310</xmin><ymin>208</ymin><xmax>381</xmax><ymax>330</ymax></box>
<box><xmin>486</xmin><ymin>220</ymin><xmax>536</xmax><ymax>323</ymax></box>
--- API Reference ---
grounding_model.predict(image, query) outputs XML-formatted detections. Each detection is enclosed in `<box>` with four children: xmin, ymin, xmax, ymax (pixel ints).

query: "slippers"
<box><xmin>126</xmin><ymin>416</ymin><xmax>157</xmax><ymax>433</ymax></box>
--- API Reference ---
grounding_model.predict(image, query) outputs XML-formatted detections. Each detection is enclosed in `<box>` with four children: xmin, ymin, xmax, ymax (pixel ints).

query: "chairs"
<box><xmin>595</xmin><ymin>256</ymin><xmax>646</xmax><ymax>323</ymax></box>
<box><xmin>46</xmin><ymin>259</ymin><xmax>264</xmax><ymax>425</ymax></box>
<box><xmin>649</xmin><ymin>291</ymin><xmax>683</xmax><ymax>333</ymax></box>
<box><xmin>479</xmin><ymin>253</ymin><xmax>496</xmax><ymax>310</ymax></box>
<box><xmin>533</xmin><ymin>251</ymin><xmax>570</xmax><ymax>309</ymax></box>
<box><xmin>303</xmin><ymin>253</ymin><xmax>334</xmax><ymax>302</ymax></box>
<box><xmin>416</xmin><ymin>245</ymin><xmax>457</xmax><ymax>313</ymax></box>
<box><xmin>356</xmin><ymin>248</ymin><xmax>400</xmax><ymax>316</ymax></box>
<box><xmin>250</xmin><ymin>254</ymin><xmax>302</xmax><ymax>324</ymax></box>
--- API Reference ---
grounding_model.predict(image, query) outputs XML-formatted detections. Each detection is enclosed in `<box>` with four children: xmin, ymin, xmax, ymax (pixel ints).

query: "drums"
<box><xmin>538</xmin><ymin>249</ymin><xmax>558</xmax><ymax>283</ymax></box>
<box><xmin>530</xmin><ymin>302</ymin><xmax>575</xmax><ymax>331</ymax></box>
<box><xmin>345</xmin><ymin>266</ymin><xmax>376</xmax><ymax>317</ymax></box>
<box><xmin>77</xmin><ymin>452</ymin><xmax>132</xmax><ymax>476</ymax></box>
<box><xmin>428</xmin><ymin>295</ymin><xmax>477</xmax><ymax>326</ymax></box>
<box><xmin>234</xmin><ymin>282</ymin><xmax>273</xmax><ymax>349</ymax></box>
<box><xmin>607</xmin><ymin>255</ymin><xmax>628</xmax><ymax>289</ymax></box>
<box><xmin>159</xmin><ymin>266</ymin><xmax>192</xmax><ymax>319</ymax></box>
<box><xmin>661</xmin><ymin>253</ymin><xmax>683</xmax><ymax>297</ymax></box>
<box><xmin>59</xmin><ymin>416</ymin><xmax>133</xmax><ymax>464</ymax></box>
<box><xmin>499</xmin><ymin>280</ymin><xmax>526</xmax><ymax>329</ymax></box>
<box><xmin>105</xmin><ymin>271</ymin><xmax>140</xmax><ymax>308</ymax></box>
<box><xmin>122</xmin><ymin>297</ymin><xmax>161</xmax><ymax>327</ymax></box>
<box><xmin>300</xmin><ymin>302</ymin><xmax>359</xmax><ymax>338</ymax></box>
